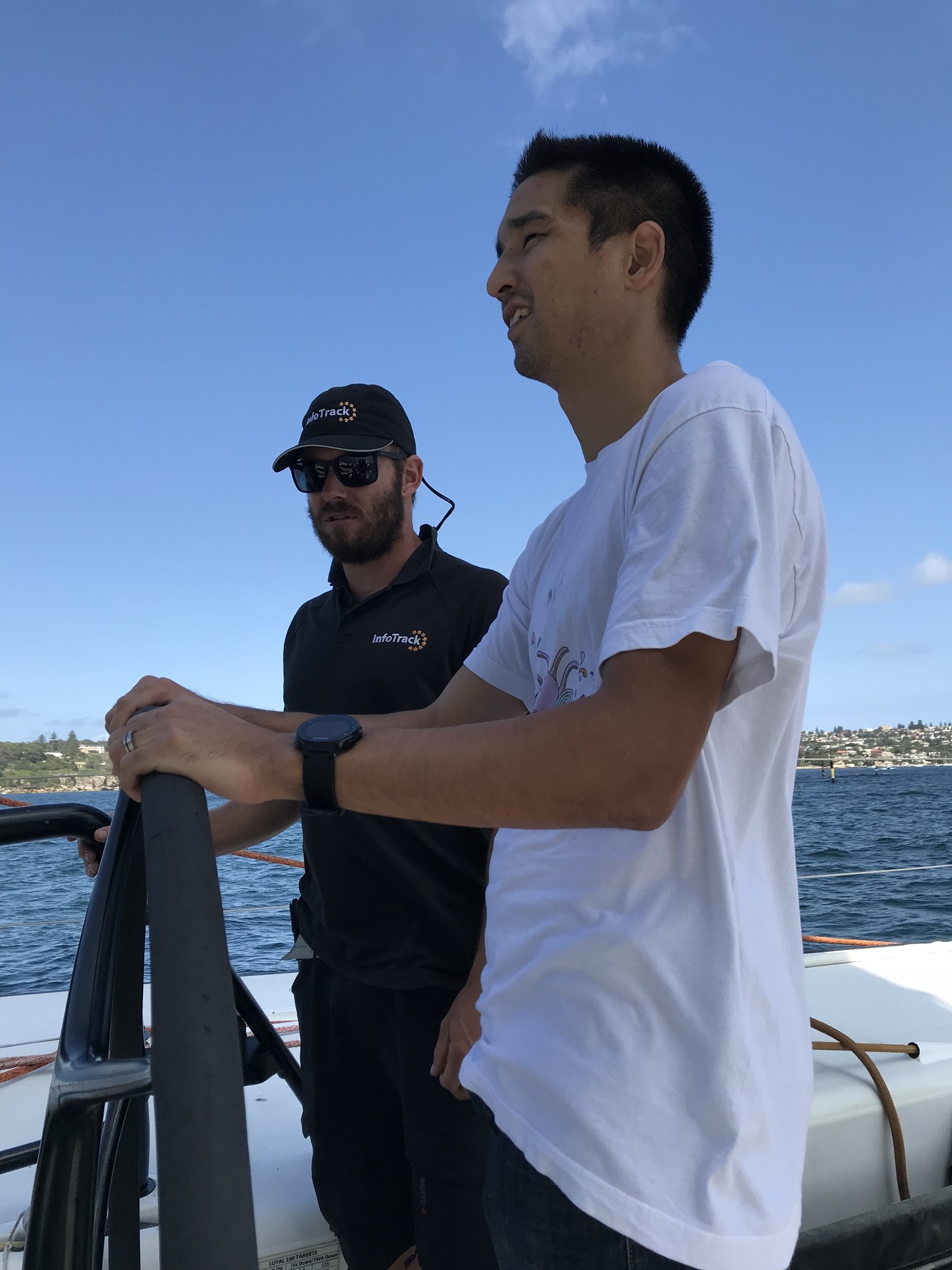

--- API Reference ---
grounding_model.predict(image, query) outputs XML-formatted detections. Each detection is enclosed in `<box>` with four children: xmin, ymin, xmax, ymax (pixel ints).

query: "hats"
<box><xmin>272</xmin><ymin>384</ymin><xmax>415</xmax><ymax>473</ymax></box>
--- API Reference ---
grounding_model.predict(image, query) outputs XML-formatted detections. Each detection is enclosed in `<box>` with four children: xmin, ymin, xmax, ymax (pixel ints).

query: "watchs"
<box><xmin>291</xmin><ymin>714</ymin><xmax>363</xmax><ymax>822</ymax></box>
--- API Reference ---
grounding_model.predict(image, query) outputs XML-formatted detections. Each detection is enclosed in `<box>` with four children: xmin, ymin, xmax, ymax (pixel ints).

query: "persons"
<box><xmin>71</xmin><ymin>384</ymin><xmax>508</xmax><ymax>1267</ymax></box>
<box><xmin>97</xmin><ymin>127</ymin><xmax>821</xmax><ymax>1269</ymax></box>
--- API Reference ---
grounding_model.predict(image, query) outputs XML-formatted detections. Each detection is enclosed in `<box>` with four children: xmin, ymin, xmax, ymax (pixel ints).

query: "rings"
<box><xmin>122</xmin><ymin>732</ymin><xmax>137</xmax><ymax>753</ymax></box>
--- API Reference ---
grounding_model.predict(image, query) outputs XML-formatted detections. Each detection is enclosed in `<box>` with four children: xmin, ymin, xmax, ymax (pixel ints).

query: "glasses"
<box><xmin>286</xmin><ymin>449</ymin><xmax>412</xmax><ymax>492</ymax></box>
